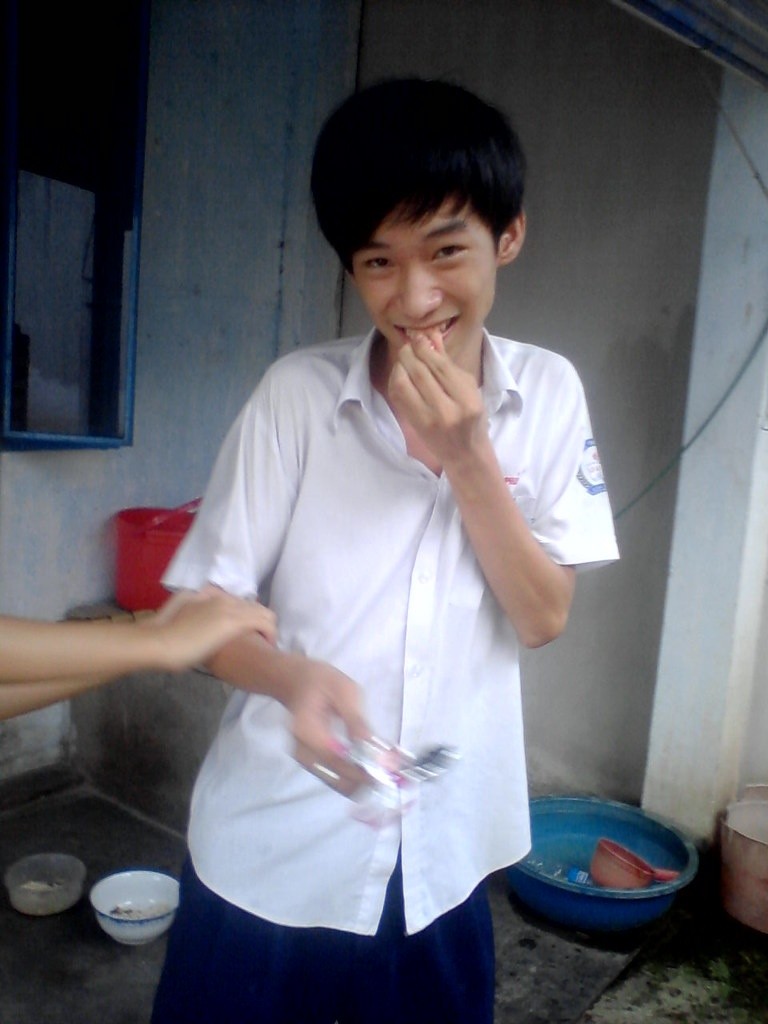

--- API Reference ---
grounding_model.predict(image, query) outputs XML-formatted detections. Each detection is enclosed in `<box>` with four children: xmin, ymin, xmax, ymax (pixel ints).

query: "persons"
<box><xmin>0</xmin><ymin>585</ymin><xmax>277</xmax><ymax>721</ymax></box>
<box><xmin>150</xmin><ymin>73</ymin><xmax>621</xmax><ymax>1024</ymax></box>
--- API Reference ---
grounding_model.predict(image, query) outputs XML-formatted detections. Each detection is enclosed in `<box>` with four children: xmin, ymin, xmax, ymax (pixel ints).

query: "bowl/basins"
<box><xmin>507</xmin><ymin>795</ymin><xmax>698</xmax><ymax>948</ymax></box>
<box><xmin>90</xmin><ymin>870</ymin><xmax>179</xmax><ymax>945</ymax></box>
<box><xmin>4</xmin><ymin>854</ymin><xmax>85</xmax><ymax>914</ymax></box>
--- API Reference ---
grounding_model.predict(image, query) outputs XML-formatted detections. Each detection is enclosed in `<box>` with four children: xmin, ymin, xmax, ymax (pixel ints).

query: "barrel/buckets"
<box><xmin>721</xmin><ymin>784</ymin><xmax>768</xmax><ymax>933</ymax></box>
<box><xmin>116</xmin><ymin>498</ymin><xmax>202</xmax><ymax>611</ymax></box>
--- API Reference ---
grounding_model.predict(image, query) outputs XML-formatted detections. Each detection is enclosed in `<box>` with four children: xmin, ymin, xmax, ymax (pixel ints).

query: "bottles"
<box><xmin>528</xmin><ymin>858</ymin><xmax>594</xmax><ymax>887</ymax></box>
